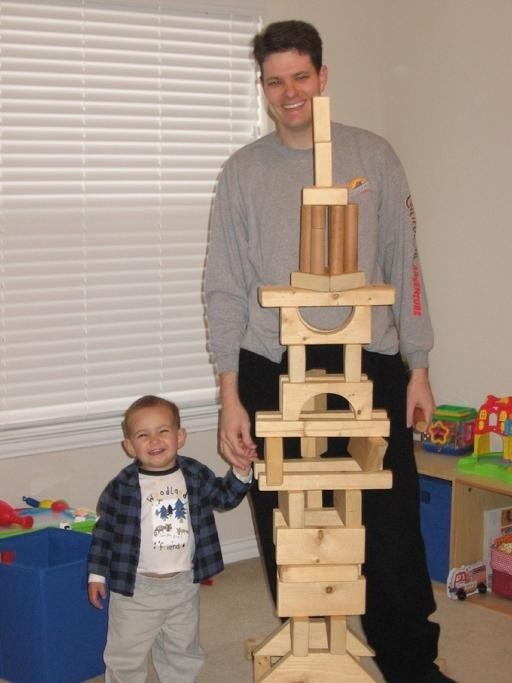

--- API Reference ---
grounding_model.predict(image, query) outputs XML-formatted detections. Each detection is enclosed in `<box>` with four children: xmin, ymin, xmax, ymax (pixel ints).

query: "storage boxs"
<box><xmin>423</xmin><ymin>403</ymin><xmax>479</xmax><ymax>454</ymax></box>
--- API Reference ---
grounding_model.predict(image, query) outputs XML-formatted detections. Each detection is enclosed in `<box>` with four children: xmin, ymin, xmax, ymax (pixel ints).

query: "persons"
<box><xmin>87</xmin><ymin>396</ymin><xmax>256</xmax><ymax>682</ymax></box>
<box><xmin>201</xmin><ymin>18</ymin><xmax>453</xmax><ymax>682</ymax></box>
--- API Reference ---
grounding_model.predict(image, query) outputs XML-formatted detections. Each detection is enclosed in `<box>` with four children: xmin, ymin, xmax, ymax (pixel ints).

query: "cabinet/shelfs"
<box><xmin>411</xmin><ymin>439</ymin><xmax>512</xmax><ymax>618</ymax></box>
